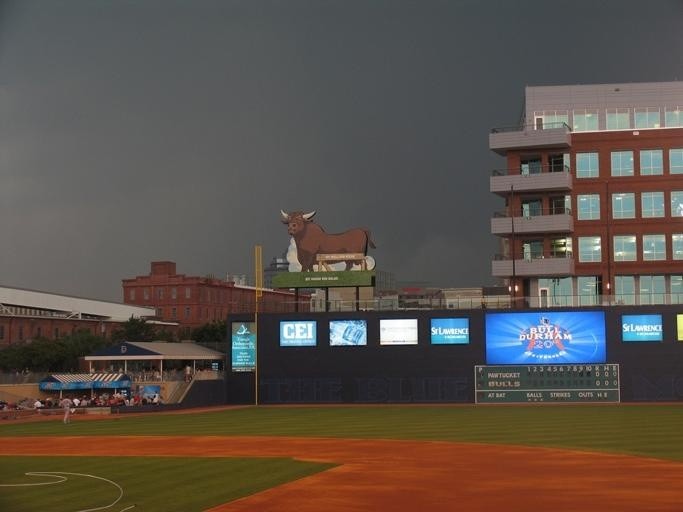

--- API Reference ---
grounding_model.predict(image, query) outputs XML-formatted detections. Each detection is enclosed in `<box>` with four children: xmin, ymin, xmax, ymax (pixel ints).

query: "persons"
<box><xmin>480</xmin><ymin>296</ymin><xmax>486</xmax><ymax>309</ymax></box>
<box><xmin>0</xmin><ymin>362</ymin><xmax>212</xmax><ymax>424</ymax></box>
